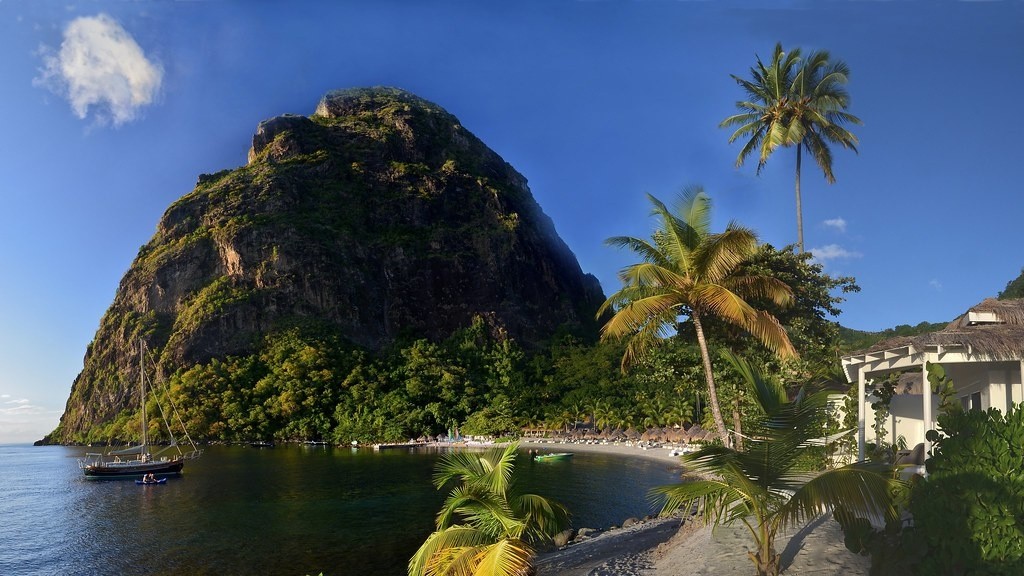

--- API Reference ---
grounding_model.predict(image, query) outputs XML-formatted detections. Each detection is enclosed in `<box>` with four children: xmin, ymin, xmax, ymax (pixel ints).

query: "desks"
<box><xmin>893</xmin><ymin>449</ymin><xmax>913</xmax><ymax>454</ymax></box>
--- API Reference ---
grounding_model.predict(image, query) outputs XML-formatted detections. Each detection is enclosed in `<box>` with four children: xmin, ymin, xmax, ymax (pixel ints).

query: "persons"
<box><xmin>143</xmin><ymin>473</ymin><xmax>157</xmax><ymax>484</ymax></box>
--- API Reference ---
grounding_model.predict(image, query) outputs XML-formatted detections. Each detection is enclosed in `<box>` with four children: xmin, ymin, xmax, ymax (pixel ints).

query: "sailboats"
<box><xmin>76</xmin><ymin>335</ymin><xmax>207</xmax><ymax>482</ymax></box>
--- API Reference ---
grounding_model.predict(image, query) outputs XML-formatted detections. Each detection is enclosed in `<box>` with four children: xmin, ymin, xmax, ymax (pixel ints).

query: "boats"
<box><xmin>134</xmin><ymin>477</ymin><xmax>167</xmax><ymax>485</ymax></box>
<box><xmin>533</xmin><ymin>452</ymin><xmax>574</xmax><ymax>462</ymax></box>
<box><xmin>249</xmin><ymin>441</ymin><xmax>276</xmax><ymax>447</ymax></box>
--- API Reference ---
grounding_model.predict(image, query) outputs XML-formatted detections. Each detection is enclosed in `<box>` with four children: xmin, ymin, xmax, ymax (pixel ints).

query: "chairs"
<box><xmin>897</xmin><ymin>443</ymin><xmax>926</xmax><ymax>467</ymax></box>
